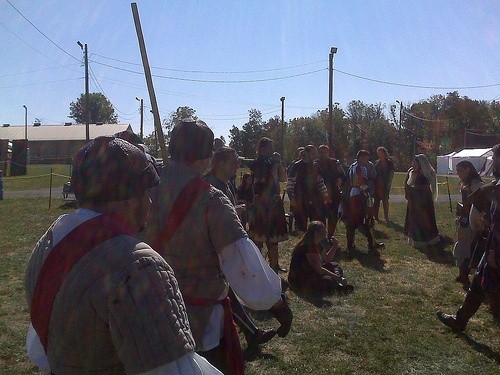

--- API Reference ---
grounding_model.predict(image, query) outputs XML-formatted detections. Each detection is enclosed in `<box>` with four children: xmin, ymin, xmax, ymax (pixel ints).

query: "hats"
<box><xmin>72</xmin><ymin>136</ymin><xmax>160</xmax><ymax>203</ymax></box>
<box><xmin>168</xmin><ymin>118</ymin><xmax>215</xmax><ymax>158</ymax></box>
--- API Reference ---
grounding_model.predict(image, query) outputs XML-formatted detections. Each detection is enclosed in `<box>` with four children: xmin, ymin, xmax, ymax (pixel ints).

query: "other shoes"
<box><xmin>347</xmin><ymin>239</ymin><xmax>385</xmax><ymax>257</ymax></box>
<box><xmin>336</xmin><ymin>282</ymin><xmax>353</xmax><ymax>294</ymax></box>
<box><xmin>374</xmin><ymin>218</ymin><xmax>389</xmax><ymax>225</ymax></box>
<box><xmin>270</xmin><ymin>265</ymin><xmax>287</xmax><ymax>274</ymax></box>
<box><xmin>457</xmin><ymin>267</ymin><xmax>470</xmax><ymax>291</ymax></box>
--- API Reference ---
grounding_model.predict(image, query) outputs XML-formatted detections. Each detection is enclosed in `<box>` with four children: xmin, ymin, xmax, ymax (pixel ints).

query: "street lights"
<box><xmin>280</xmin><ymin>96</ymin><xmax>287</xmax><ymax>163</ymax></box>
<box><xmin>150</xmin><ymin>109</ymin><xmax>158</xmax><ymax>160</ymax></box>
<box><xmin>21</xmin><ymin>104</ymin><xmax>27</xmax><ymax>142</ymax></box>
<box><xmin>135</xmin><ymin>97</ymin><xmax>143</xmax><ymax>144</ymax></box>
<box><xmin>396</xmin><ymin>99</ymin><xmax>404</xmax><ymax>172</ymax></box>
<box><xmin>328</xmin><ymin>46</ymin><xmax>337</xmax><ymax>159</ymax></box>
<box><xmin>77</xmin><ymin>40</ymin><xmax>91</xmax><ymax>145</ymax></box>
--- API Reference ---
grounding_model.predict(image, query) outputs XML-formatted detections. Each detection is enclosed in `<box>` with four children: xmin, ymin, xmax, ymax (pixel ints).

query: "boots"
<box><xmin>436</xmin><ymin>287</ymin><xmax>485</xmax><ymax>332</ymax></box>
<box><xmin>491</xmin><ymin>348</ymin><xmax>500</xmax><ymax>362</ymax></box>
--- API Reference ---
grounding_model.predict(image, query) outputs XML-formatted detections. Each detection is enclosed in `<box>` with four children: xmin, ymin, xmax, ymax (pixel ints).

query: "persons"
<box><xmin>248</xmin><ymin>137</ymin><xmax>289</xmax><ymax>274</ymax></box>
<box><xmin>204</xmin><ymin>146</ymin><xmax>278</xmax><ymax>348</ymax></box>
<box><xmin>436</xmin><ymin>144</ymin><xmax>500</xmax><ymax>335</ymax></box>
<box><xmin>465</xmin><ymin>178</ymin><xmax>495</xmax><ymax>235</ymax></box>
<box><xmin>136</xmin><ymin>118</ymin><xmax>295</xmax><ymax>375</ymax></box>
<box><xmin>284</xmin><ymin>144</ymin><xmax>443</xmax><ymax>260</ymax></box>
<box><xmin>453</xmin><ymin>160</ymin><xmax>487</xmax><ymax>284</ymax></box>
<box><xmin>20</xmin><ymin>137</ymin><xmax>223</xmax><ymax>375</ymax></box>
<box><xmin>287</xmin><ymin>218</ymin><xmax>353</xmax><ymax>297</ymax></box>
<box><xmin>237</xmin><ymin>171</ymin><xmax>254</xmax><ymax>232</ymax></box>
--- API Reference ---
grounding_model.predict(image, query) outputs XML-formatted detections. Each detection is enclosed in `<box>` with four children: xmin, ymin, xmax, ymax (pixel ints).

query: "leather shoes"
<box><xmin>245</xmin><ymin>328</ymin><xmax>276</xmax><ymax>348</ymax></box>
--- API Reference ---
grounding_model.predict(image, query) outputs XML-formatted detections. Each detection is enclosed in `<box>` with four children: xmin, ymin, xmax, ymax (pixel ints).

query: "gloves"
<box><xmin>270</xmin><ymin>293</ymin><xmax>293</xmax><ymax>338</ymax></box>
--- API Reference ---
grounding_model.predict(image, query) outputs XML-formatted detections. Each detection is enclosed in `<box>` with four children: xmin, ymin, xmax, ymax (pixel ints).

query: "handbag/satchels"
<box><xmin>455</xmin><ymin>202</ymin><xmax>469</xmax><ymax>227</ymax></box>
